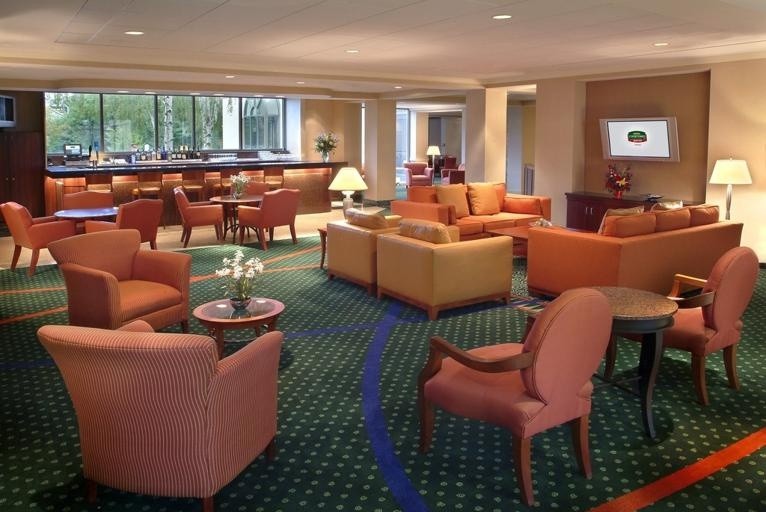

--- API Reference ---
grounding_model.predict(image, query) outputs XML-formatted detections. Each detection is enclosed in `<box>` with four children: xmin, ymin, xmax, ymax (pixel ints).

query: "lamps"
<box><xmin>709</xmin><ymin>160</ymin><xmax>752</xmax><ymax>220</ymax></box>
<box><xmin>426</xmin><ymin>145</ymin><xmax>441</xmax><ymax>168</ymax></box>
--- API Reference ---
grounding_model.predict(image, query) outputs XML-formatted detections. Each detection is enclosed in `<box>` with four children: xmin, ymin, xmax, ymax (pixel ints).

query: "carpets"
<box><xmin>0</xmin><ymin>234</ymin><xmax>766</xmax><ymax>512</ymax></box>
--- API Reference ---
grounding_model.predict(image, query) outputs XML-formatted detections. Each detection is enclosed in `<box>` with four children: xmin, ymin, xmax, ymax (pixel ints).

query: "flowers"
<box><xmin>312</xmin><ymin>128</ymin><xmax>339</xmax><ymax>160</ymax></box>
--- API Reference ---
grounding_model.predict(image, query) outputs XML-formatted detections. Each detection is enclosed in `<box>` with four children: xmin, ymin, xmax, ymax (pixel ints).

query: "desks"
<box><xmin>596</xmin><ymin>287</ymin><xmax>680</xmax><ymax>441</ymax></box>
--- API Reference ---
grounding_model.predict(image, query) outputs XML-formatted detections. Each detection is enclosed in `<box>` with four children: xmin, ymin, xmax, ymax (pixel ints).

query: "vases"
<box><xmin>320</xmin><ymin>152</ymin><xmax>329</xmax><ymax>164</ymax></box>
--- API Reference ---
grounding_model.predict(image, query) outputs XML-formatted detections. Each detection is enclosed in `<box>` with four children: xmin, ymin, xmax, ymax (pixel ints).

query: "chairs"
<box><xmin>36</xmin><ymin>319</ymin><xmax>283</xmax><ymax>512</ymax></box>
<box><xmin>662</xmin><ymin>247</ymin><xmax>759</xmax><ymax>407</ymax></box>
<box><xmin>416</xmin><ymin>286</ymin><xmax>613</xmax><ymax>506</ymax></box>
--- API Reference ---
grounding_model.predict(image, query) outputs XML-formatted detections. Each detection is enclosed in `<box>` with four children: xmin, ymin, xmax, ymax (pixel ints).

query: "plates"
<box><xmin>639</xmin><ymin>194</ymin><xmax>664</xmax><ymax>200</ymax></box>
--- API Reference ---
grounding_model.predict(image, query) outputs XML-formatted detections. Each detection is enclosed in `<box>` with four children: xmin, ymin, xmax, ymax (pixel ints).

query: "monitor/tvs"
<box><xmin>598</xmin><ymin>117</ymin><xmax>681</xmax><ymax>163</ymax></box>
<box><xmin>0</xmin><ymin>94</ymin><xmax>18</xmax><ymax>127</ymax></box>
<box><xmin>64</xmin><ymin>143</ymin><xmax>81</xmax><ymax>158</ymax></box>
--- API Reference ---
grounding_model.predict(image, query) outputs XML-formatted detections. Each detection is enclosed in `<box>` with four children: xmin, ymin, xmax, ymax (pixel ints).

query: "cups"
<box><xmin>109</xmin><ymin>157</ymin><xmax>126</xmax><ymax>165</ymax></box>
<box><xmin>207</xmin><ymin>153</ymin><xmax>238</xmax><ymax>162</ymax></box>
<box><xmin>257</xmin><ymin>150</ymin><xmax>297</xmax><ymax>161</ymax></box>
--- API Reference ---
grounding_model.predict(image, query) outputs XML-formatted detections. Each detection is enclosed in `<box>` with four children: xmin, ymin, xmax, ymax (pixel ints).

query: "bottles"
<box><xmin>130</xmin><ymin>143</ymin><xmax>201</xmax><ymax>163</ymax></box>
<box><xmin>94</xmin><ymin>141</ymin><xmax>99</xmax><ymax>156</ymax></box>
